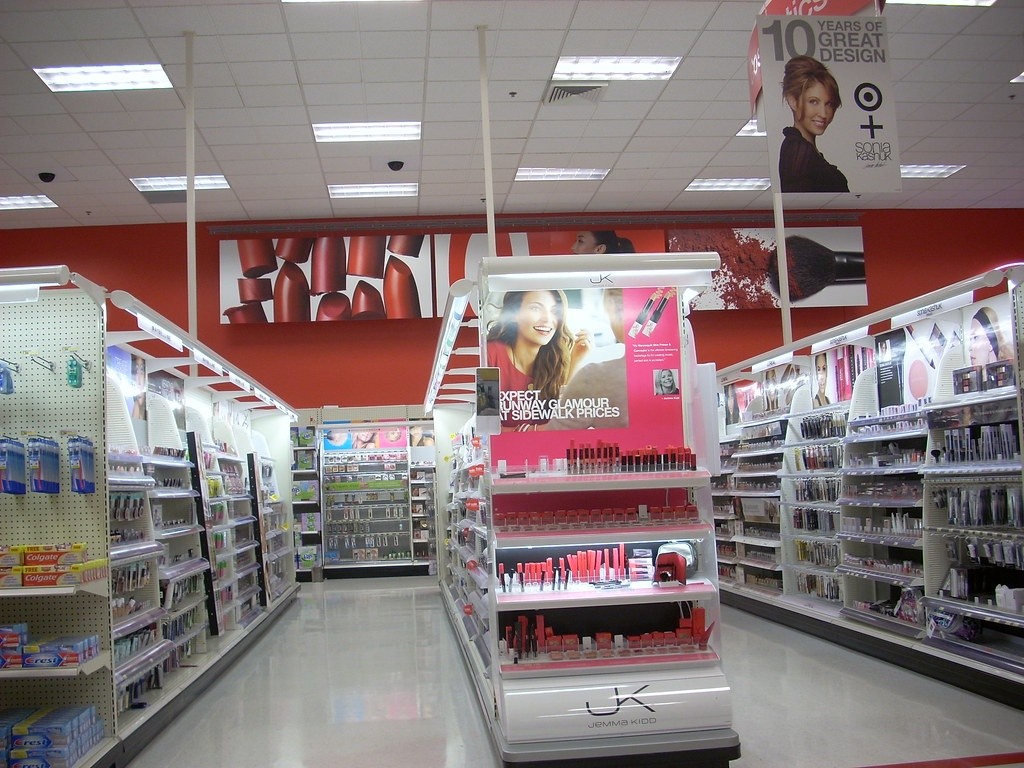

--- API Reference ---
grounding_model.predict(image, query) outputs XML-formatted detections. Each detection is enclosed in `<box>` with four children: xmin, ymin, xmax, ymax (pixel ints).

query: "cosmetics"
<box><xmin>224</xmin><ymin>232</ymin><xmax>426</xmax><ymax>324</ymax></box>
<box><xmin>106</xmin><ymin>438</ymin><xmax>289</xmax><ymax>716</ymax></box>
<box><xmin>769</xmin><ymin>234</ymin><xmax>866</xmax><ymax>305</ymax></box>
<box><xmin>291</xmin><ymin>426</ymin><xmax>435</xmax><ymax>564</ymax></box>
<box><xmin>491</xmin><ymin>440</ymin><xmax>716</xmax><ymax>664</ymax></box>
<box><xmin>712</xmin><ymin>364</ymin><xmax>1024</xmax><ymax>642</ymax></box>
<box><xmin>628</xmin><ymin>288</ymin><xmax>674</xmax><ymax>339</ymax></box>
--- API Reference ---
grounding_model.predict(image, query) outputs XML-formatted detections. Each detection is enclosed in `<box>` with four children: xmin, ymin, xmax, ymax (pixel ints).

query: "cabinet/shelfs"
<box><xmin>710</xmin><ymin>263</ymin><xmax>1024</xmax><ymax>710</ymax></box>
<box><xmin>289</xmin><ymin>421</ymin><xmax>437</xmax><ymax>582</ymax></box>
<box><xmin>423</xmin><ymin>250</ymin><xmax>741</xmax><ymax>768</ymax></box>
<box><xmin>0</xmin><ymin>265</ymin><xmax>301</xmax><ymax>768</ymax></box>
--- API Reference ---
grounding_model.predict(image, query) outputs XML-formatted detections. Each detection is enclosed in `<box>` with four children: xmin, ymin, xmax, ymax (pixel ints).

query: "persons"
<box><xmin>571</xmin><ymin>230</ymin><xmax>635</xmax><ymax>253</ymax></box>
<box><xmin>813</xmin><ymin>352</ymin><xmax>830</xmax><ymax>410</ymax></box>
<box><xmin>765</xmin><ymin>369</ymin><xmax>778</xmax><ymax>411</ymax></box>
<box><xmin>969</xmin><ymin>307</ymin><xmax>1014</xmax><ymax>366</ymax></box>
<box><xmin>351</xmin><ymin>430</ymin><xmax>375</xmax><ymax>449</ymax></box>
<box><xmin>487</xmin><ymin>288</ymin><xmax>629</xmax><ymax>430</ymax></box>
<box><xmin>131</xmin><ymin>353</ymin><xmax>146</xmax><ymax>420</ymax></box>
<box><xmin>409</xmin><ymin>426</ymin><xmax>435</xmax><ymax>446</ymax></box>
<box><xmin>779</xmin><ymin>56</ymin><xmax>850</xmax><ymax>193</ymax></box>
<box><xmin>654</xmin><ymin>369</ymin><xmax>679</xmax><ymax>396</ymax></box>
<box><xmin>724</xmin><ymin>384</ymin><xmax>739</xmax><ymax>426</ymax></box>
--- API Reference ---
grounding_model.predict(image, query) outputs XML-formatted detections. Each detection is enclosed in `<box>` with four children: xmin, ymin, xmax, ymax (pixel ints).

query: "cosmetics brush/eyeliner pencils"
<box><xmin>907</xmin><ymin>325</ymin><xmax>936</xmax><ymax>369</ymax></box>
